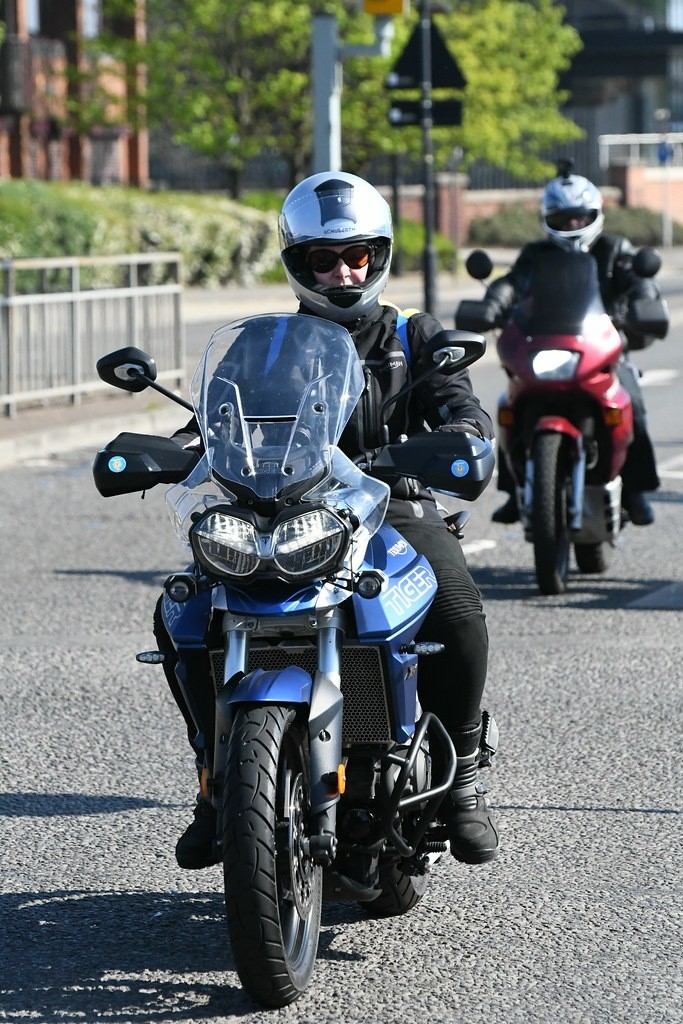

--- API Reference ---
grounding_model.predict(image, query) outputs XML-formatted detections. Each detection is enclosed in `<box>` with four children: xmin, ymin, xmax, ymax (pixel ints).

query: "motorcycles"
<box><xmin>455</xmin><ymin>249</ymin><xmax>668</xmax><ymax>594</ymax></box>
<box><xmin>94</xmin><ymin>313</ymin><xmax>495</xmax><ymax>1006</ymax></box>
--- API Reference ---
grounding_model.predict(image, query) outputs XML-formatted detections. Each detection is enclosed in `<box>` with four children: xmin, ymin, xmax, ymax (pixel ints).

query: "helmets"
<box><xmin>278</xmin><ymin>172</ymin><xmax>394</xmax><ymax>320</ymax></box>
<box><xmin>538</xmin><ymin>175</ymin><xmax>605</xmax><ymax>251</ymax></box>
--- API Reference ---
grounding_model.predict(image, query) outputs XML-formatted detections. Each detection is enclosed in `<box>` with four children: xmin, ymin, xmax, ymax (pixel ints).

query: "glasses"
<box><xmin>308</xmin><ymin>244</ymin><xmax>371</xmax><ymax>274</ymax></box>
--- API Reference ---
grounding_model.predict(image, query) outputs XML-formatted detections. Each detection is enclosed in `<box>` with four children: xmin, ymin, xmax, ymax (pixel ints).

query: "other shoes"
<box><xmin>623</xmin><ymin>496</ymin><xmax>655</xmax><ymax>524</ymax></box>
<box><xmin>490</xmin><ymin>497</ymin><xmax>521</xmax><ymax>526</ymax></box>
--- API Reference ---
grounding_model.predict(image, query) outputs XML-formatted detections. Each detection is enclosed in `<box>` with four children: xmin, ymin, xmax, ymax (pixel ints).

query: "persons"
<box><xmin>459</xmin><ymin>153</ymin><xmax>669</xmax><ymax>528</ymax></box>
<box><xmin>150</xmin><ymin>159</ymin><xmax>512</xmax><ymax>877</ymax></box>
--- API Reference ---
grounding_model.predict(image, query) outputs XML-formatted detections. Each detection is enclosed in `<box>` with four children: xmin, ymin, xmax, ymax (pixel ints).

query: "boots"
<box><xmin>443</xmin><ymin>711</ymin><xmax>500</xmax><ymax>864</ymax></box>
<box><xmin>172</xmin><ymin>760</ymin><xmax>219</xmax><ymax>872</ymax></box>
